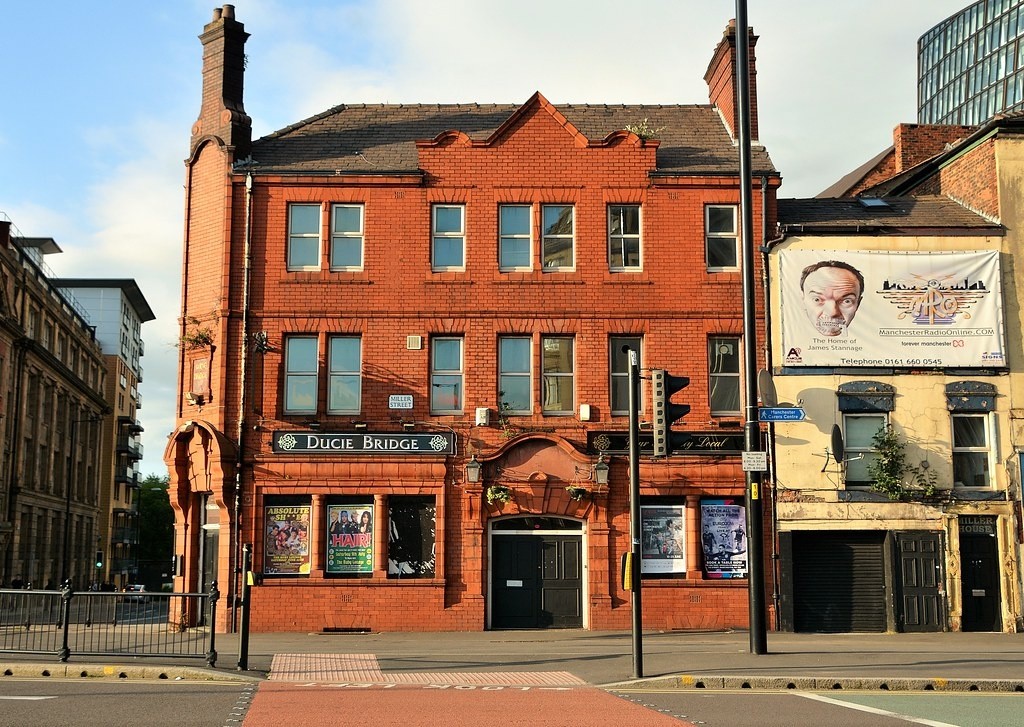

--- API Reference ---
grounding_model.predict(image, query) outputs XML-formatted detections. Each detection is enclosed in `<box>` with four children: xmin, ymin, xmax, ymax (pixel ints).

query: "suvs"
<box><xmin>100</xmin><ymin>584</ymin><xmax>119</xmax><ymax>593</ymax></box>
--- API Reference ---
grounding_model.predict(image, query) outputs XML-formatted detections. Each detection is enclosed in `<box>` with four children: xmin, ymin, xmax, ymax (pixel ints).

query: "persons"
<box><xmin>703</xmin><ymin>524</ymin><xmax>718</xmax><ymax>553</ymax></box>
<box><xmin>329</xmin><ymin>510</ymin><xmax>372</xmax><ymax>544</ymax></box>
<box><xmin>704</xmin><ymin>544</ymin><xmax>746</xmax><ymax>571</ymax></box>
<box><xmin>800</xmin><ymin>261</ymin><xmax>865</xmax><ymax>336</ymax></box>
<box><xmin>10</xmin><ymin>574</ymin><xmax>24</xmax><ymax>612</ymax></box>
<box><xmin>92</xmin><ymin>579</ymin><xmax>118</xmax><ymax>604</ymax></box>
<box><xmin>731</xmin><ymin>525</ymin><xmax>745</xmax><ymax>552</ymax></box>
<box><xmin>652</xmin><ymin>519</ymin><xmax>682</xmax><ymax>558</ymax></box>
<box><xmin>268</xmin><ymin>514</ymin><xmax>308</xmax><ymax>554</ymax></box>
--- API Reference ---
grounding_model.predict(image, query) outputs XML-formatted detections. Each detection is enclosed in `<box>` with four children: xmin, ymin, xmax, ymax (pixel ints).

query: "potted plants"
<box><xmin>486</xmin><ymin>485</ymin><xmax>510</xmax><ymax>505</ymax></box>
<box><xmin>565</xmin><ymin>486</ymin><xmax>585</xmax><ymax>500</ymax></box>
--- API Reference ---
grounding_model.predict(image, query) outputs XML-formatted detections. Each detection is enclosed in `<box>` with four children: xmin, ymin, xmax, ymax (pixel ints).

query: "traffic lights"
<box><xmin>95</xmin><ymin>551</ymin><xmax>104</xmax><ymax>570</ymax></box>
<box><xmin>652</xmin><ymin>369</ymin><xmax>692</xmax><ymax>460</ymax></box>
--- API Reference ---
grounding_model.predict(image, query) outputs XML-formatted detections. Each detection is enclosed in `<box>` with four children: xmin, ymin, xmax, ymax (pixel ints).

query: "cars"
<box><xmin>122</xmin><ymin>584</ymin><xmax>152</xmax><ymax>603</ymax></box>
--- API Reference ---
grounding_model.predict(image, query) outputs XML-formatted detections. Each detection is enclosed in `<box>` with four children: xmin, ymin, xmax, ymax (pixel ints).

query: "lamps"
<box><xmin>466</xmin><ymin>453</ymin><xmax>482</xmax><ymax>483</ymax></box>
<box><xmin>591</xmin><ymin>452</ymin><xmax>609</xmax><ymax>485</ymax></box>
<box><xmin>309</xmin><ymin>421</ymin><xmax>321</xmax><ymax>429</ymax></box>
<box><xmin>404</xmin><ymin>422</ymin><xmax>414</xmax><ymax>430</ymax></box>
<box><xmin>355</xmin><ymin>420</ymin><xmax>367</xmax><ymax>430</ymax></box>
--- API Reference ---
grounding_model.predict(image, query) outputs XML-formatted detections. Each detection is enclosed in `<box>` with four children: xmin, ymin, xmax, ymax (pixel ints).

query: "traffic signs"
<box><xmin>759</xmin><ymin>409</ymin><xmax>807</xmax><ymax>422</ymax></box>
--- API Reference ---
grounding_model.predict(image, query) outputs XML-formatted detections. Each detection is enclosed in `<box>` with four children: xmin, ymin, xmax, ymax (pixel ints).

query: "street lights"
<box><xmin>132</xmin><ymin>487</ymin><xmax>162</xmax><ymax>584</ymax></box>
